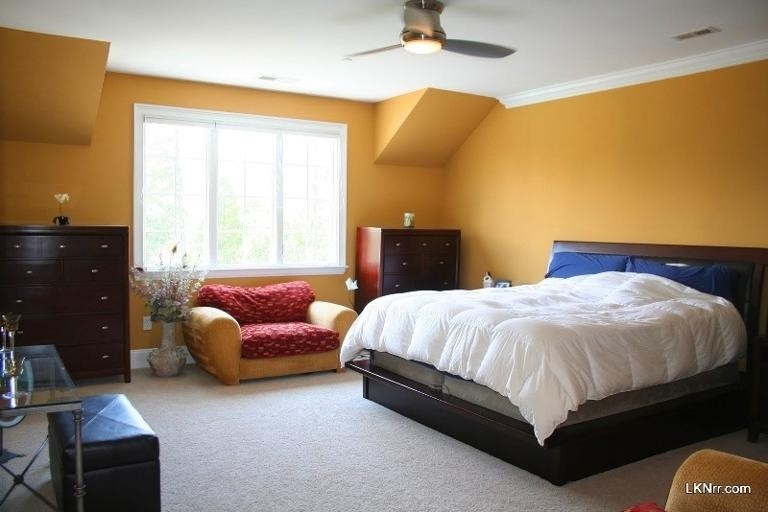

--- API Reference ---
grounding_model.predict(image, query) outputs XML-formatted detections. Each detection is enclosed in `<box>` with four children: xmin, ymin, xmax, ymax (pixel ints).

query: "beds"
<box><xmin>346</xmin><ymin>241</ymin><xmax>768</xmax><ymax>487</ymax></box>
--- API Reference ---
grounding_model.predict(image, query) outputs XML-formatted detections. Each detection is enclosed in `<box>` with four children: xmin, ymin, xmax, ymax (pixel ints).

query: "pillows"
<box><xmin>196</xmin><ymin>280</ymin><xmax>315</xmax><ymax>324</ymax></box>
<box><xmin>627</xmin><ymin>255</ymin><xmax>739</xmax><ymax>303</ymax></box>
<box><xmin>543</xmin><ymin>251</ymin><xmax>629</xmax><ymax>279</ymax></box>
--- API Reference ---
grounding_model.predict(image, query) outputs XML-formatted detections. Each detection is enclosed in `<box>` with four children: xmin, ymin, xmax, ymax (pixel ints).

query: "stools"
<box><xmin>46</xmin><ymin>391</ymin><xmax>161</xmax><ymax>512</ymax></box>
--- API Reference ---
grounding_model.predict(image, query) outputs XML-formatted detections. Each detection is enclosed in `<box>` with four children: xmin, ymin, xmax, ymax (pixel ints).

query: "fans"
<box><xmin>347</xmin><ymin>0</ymin><xmax>520</xmax><ymax>60</ymax></box>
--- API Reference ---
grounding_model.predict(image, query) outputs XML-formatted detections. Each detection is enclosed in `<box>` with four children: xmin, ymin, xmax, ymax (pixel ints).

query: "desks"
<box><xmin>0</xmin><ymin>344</ymin><xmax>88</xmax><ymax>512</ymax></box>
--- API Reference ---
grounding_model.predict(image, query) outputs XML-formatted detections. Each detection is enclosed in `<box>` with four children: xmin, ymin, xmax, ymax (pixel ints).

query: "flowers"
<box><xmin>51</xmin><ymin>192</ymin><xmax>71</xmax><ymax>225</ymax></box>
<box><xmin>129</xmin><ymin>242</ymin><xmax>206</xmax><ymax>323</ymax></box>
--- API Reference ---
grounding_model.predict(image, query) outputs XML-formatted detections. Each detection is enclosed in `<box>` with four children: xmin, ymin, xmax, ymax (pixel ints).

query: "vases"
<box><xmin>146</xmin><ymin>320</ymin><xmax>188</xmax><ymax>378</ymax></box>
<box><xmin>55</xmin><ymin>218</ymin><xmax>70</xmax><ymax>227</ymax></box>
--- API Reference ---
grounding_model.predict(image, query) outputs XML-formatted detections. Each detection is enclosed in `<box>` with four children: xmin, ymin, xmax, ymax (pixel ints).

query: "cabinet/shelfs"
<box><xmin>0</xmin><ymin>224</ymin><xmax>131</xmax><ymax>383</ymax></box>
<box><xmin>353</xmin><ymin>225</ymin><xmax>462</xmax><ymax>316</ymax></box>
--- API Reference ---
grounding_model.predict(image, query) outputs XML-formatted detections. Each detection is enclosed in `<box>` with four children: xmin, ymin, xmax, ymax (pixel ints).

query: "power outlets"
<box><xmin>142</xmin><ymin>316</ymin><xmax>153</xmax><ymax>331</ymax></box>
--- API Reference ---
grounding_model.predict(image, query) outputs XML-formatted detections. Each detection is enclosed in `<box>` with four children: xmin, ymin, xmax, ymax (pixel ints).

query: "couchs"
<box><xmin>181</xmin><ymin>301</ymin><xmax>359</xmax><ymax>386</ymax></box>
<box><xmin>665</xmin><ymin>448</ymin><xmax>767</xmax><ymax>512</ymax></box>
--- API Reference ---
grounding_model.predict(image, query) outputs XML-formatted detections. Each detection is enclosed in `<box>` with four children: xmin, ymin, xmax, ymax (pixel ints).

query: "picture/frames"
<box><xmin>493</xmin><ymin>279</ymin><xmax>511</xmax><ymax>288</ymax></box>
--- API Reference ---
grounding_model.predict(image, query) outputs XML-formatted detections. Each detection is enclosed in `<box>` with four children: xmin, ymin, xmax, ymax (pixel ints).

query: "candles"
<box><xmin>403</xmin><ymin>212</ymin><xmax>415</xmax><ymax>228</ymax></box>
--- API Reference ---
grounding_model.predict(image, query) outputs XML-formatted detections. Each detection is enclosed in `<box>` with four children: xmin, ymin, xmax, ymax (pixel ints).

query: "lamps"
<box><xmin>400</xmin><ymin>31</ymin><xmax>445</xmax><ymax>55</ymax></box>
